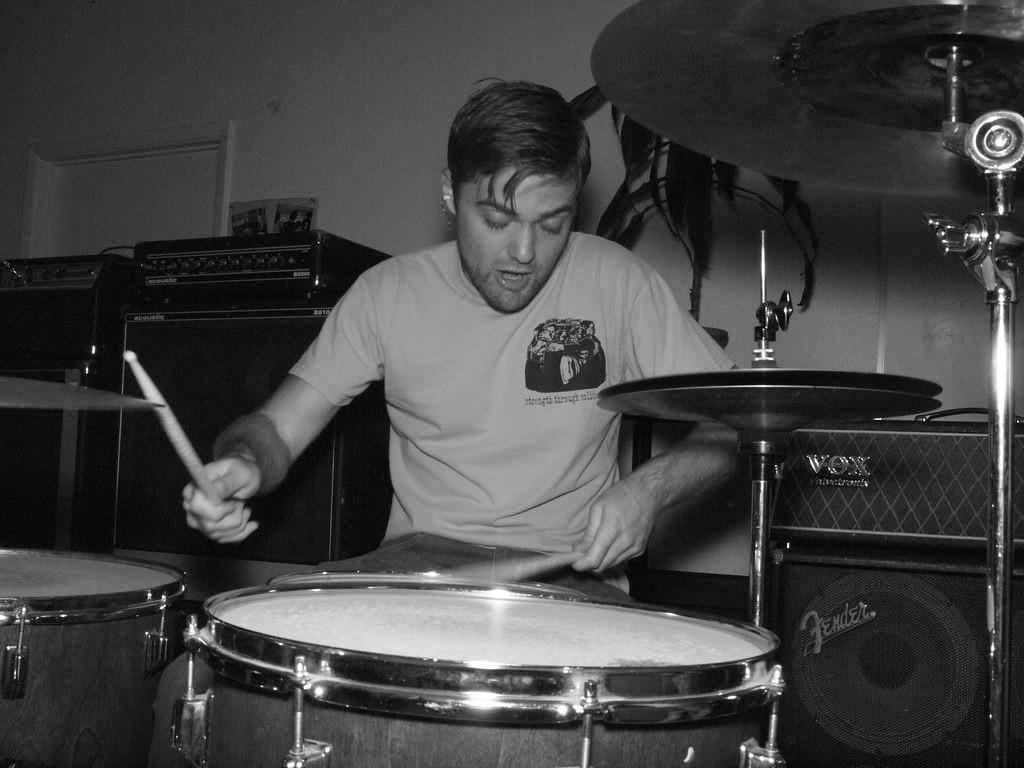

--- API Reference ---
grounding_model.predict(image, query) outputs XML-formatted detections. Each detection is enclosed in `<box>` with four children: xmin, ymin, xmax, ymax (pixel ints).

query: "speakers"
<box><xmin>768</xmin><ymin>541</ymin><xmax>1024</xmax><ymax>768</ymax></box>
<box><xmin>0</xmin><ymin>251</ymin><xmax>391</xmax><ymax>560</ymax></box>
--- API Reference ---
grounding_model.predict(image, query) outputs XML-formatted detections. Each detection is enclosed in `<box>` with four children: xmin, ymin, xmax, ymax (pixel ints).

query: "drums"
<box><xmin>0</xmin><ymin>547</ymin><xmax>187</xmax><ymax>768</ymax></box>
<box><xmin>174</xmin><ymin>580</ymin><xmax>787</xmax><ymax>768</ymax></box>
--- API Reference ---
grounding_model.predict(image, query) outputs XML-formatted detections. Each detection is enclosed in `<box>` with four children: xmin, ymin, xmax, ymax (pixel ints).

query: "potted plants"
<box><xmin>569</xmin><ymin>85</ymin><xmax>819</xmax><ymax>350</ymax></box>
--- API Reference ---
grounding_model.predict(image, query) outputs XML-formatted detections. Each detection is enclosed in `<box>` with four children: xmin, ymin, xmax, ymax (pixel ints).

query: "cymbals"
<box><xmin>586</xmin><ymin>2</ymin><xmax>1024</xmax><ymax>204</ymax></box>
<box><xmin>596</xmin><ymin>363</ymin><xmax>944</xmax><ymax>434</ymax></box>
<box><xmin>2</xmin><ymin>375</ymin><xmax>168</xmax><ymax>412</ymax></box>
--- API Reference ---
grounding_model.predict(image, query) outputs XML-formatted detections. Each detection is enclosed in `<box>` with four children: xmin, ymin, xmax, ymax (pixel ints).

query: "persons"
<box><xmin>148</xmin><ymin>76</ymin><xmax>757</xmax><ymax>768</ymax></box>
